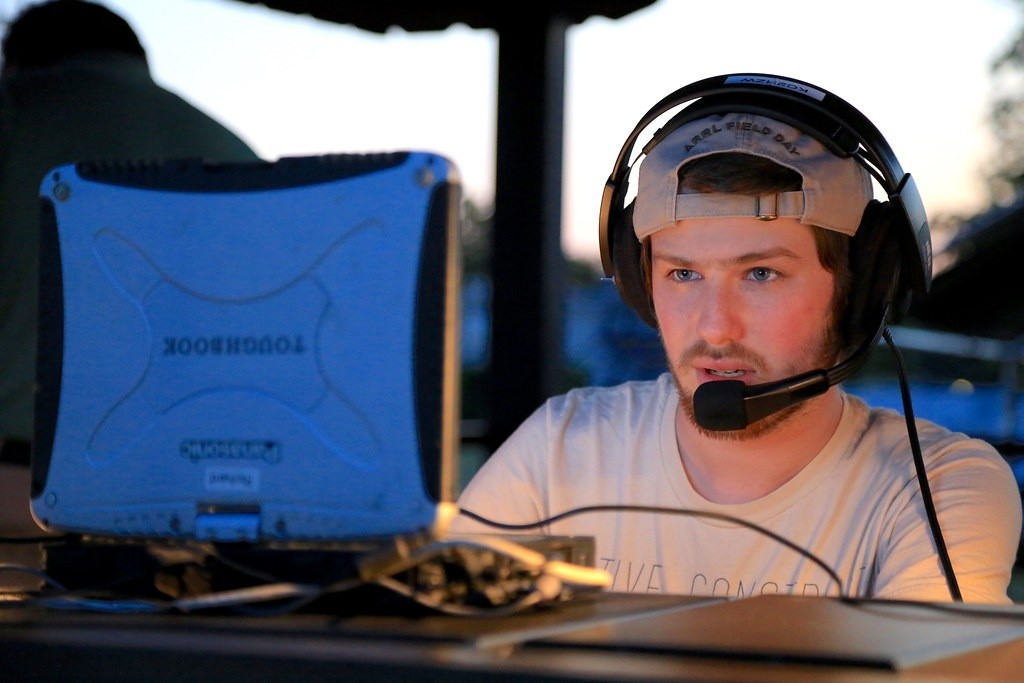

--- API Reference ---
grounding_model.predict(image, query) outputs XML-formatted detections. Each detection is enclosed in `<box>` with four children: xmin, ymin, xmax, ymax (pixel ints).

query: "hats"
<box><xmin>633</xmin><ymin>113</ymin><xmax>875</xmax><ymax>243</ymax></box>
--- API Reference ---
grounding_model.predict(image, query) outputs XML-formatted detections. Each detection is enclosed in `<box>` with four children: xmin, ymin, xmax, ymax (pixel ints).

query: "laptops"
<box><xmin>28</xmin><ymin>149</ymin><xmax>599</xmax><ymax>614</ymax></box>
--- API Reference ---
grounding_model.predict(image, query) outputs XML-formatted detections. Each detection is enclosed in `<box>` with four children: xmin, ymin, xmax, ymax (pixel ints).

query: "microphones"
<box><xmin>694</xmin><ymin>304</ymin><xmax>893</xmax><ymax>431</ymax></box>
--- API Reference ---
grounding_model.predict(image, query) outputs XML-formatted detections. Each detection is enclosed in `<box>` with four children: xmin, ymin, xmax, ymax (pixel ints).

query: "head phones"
<box><xmin>599</xmin><ymin>73</ymin><xmax>933</xmax><ymax>338</ymax></box>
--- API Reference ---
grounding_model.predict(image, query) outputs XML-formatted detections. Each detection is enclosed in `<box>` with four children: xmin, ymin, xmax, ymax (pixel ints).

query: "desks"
<box><xmin>0</xmin><ymin>576</ymin><xmax>1024</xmax><ymax>683</ymax></box>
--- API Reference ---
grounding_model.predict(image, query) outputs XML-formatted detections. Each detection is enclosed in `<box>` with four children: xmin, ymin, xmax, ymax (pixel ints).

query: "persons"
<box><xmin>0</xmin><ymin>0</ymin><xmax>260</xmax><ymax>470</ymax></box>
<box><xmin>445</xmin><ymin>73</ymin><xmax>1024</xmax><ymax>607</ymax></box>
